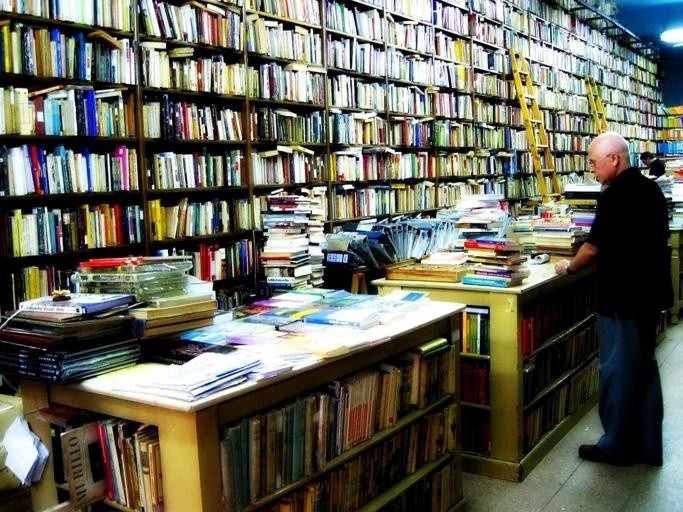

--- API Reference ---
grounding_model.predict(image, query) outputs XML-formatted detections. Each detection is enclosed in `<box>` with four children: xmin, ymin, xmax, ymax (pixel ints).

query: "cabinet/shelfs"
<box><xmin>368</xmin><ymin>243</ymin><xmax>602</xmax><ymax>484</ymax></box>
<box><xmin>0</xmin><ymin>1</ymin><xmax>659</xmax><ymax>309</ymax></box>
<box><xmin>21</xmin><ymin>286</ymin><xmax>466</xmax><ymax>511</ymax></box>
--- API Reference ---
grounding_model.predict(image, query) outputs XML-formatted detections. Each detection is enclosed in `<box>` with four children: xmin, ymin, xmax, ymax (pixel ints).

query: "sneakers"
<box><xmin>578</xmin><ymin>444</ymin><xmax>633</xmax><ymax>466</ymax></box>
<box><xmin>633</xmin><ymin>451</ymin><xmax>661</xmax><ymax>467</ymax></box>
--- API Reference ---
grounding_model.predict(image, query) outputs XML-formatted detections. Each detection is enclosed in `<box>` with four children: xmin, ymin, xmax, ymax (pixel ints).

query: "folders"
<box><xmin>322</xmin><ymin>213</ymin><xmax>455</xmax><ymax>269</ymax></box>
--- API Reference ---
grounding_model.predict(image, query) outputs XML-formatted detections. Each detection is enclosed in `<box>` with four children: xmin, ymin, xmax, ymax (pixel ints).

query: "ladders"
<box><xmin>509</xmin><ymin>47</ymin><xmax>562</xmax><ymax>202</ymax></box>
<box><xmin>585</xmin><ymin>75</ymin><xmax>608</xmax><ymax>133</ymax></box>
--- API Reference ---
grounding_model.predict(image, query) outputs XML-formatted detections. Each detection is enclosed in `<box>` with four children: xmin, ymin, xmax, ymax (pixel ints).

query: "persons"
<box><xmin>556</xmin><ymin>132</ymin><xmax>672</xmax><ymax>468</ymax></box>
<box><xmin>640</xmin><ymin>152</ymin><xmax>665</xmax><ymax>177</ymax></box>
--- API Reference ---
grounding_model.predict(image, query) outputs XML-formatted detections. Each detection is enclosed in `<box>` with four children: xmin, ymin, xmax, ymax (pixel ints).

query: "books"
<box><xmin>657</xmin><ymin>115</ymin><xmax>681</xmax><ymax>158</ymax></box>
<box><xmin>654</xmin><ymin>159</ymin><xmax>682</xmax><ymax>230</ymax></box>
<box><xmin>0</xmin><ymin>0</ymin><xmax>141</xmax><ymax>311</ymax></box>
<box><xmin>512</xmin><ymin>1</ymin><xmax>657</xmax><ymax>203</ymax></box>
<box><xmin>459</xmin><ymin>307</ymin><xmax>492</xmax><ymax>456</ymax></box>
<box><xmin>329</xmin><ymin>203</ymin><xmax>595</xmax><ymax>289</ymax></box>
<box><xmin>246</xmin><ymin>1</ymin><xmax>333</xmax><ymax>295</ymax></box>
<box><xmin>136</xmin><ymin>1</ymin><xmax>253</xmax><ymax>308</ymax></box>
<box><xmin>333</xmin><ymin>1</ymin><xmax>511</xmax><ymax>225</ymax></box>
<box><xmin>217</xmin><ymin>327</ymin><xmax>450</xmax><ymax>512</ymax></box>
<box><xmin>48</xmin><ymin>405</ymin><xmax>164</xmax><ymax>512</ymax></box>
<box><xmin>516</xmin><ymin>277</ymin><xmax>601</xmax><ymax>457</ymax></box>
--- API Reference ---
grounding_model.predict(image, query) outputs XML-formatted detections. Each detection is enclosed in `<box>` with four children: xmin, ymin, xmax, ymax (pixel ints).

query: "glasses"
<box><xmin>588</xmin><ymin>152</ymin><xmax>612</xmax><ymax>169</ymax></box>
